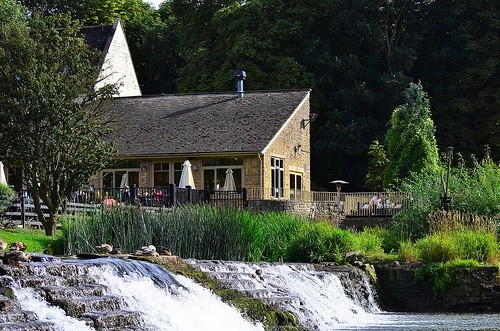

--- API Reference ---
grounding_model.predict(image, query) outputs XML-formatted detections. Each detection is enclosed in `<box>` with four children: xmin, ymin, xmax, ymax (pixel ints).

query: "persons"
<box><xmin>70</xmin><ymin>185</ymin><xmax>169</xmax><ymax>208</ymax></box>
<box><xmin>19</xmin><ymin>187</ymin><xmax>30</xmax><ymax>204</ymax></box>
<box><xmin>382</xmin><ymin>195</ymin><xmax>413</xmax><ymax>210</ymax></box>
<box><xmin>369</xmin><ymin>194</ymin><xmax>384</xmax><ymax>209</ymax></box>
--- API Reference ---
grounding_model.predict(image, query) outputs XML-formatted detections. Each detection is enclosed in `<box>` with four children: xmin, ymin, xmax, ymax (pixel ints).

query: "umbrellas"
<box><xmin>179</xmin><ymin>159</ymin><xmax>197</xmax><ymax>190</ymax></box>
<box><xmin>119</xmin><ymin>174</ymin><xmax>129</xmax><ymax>191</ymax></box>
<box><xmin>0</xmin><ymin>161</ymin><xmax>8</xmax><ymax>188</ymax></box>
<box><xmin>223</xmin><ymin>168</ymin><xmax>238</xmax><ymax>192</ymax></box>
